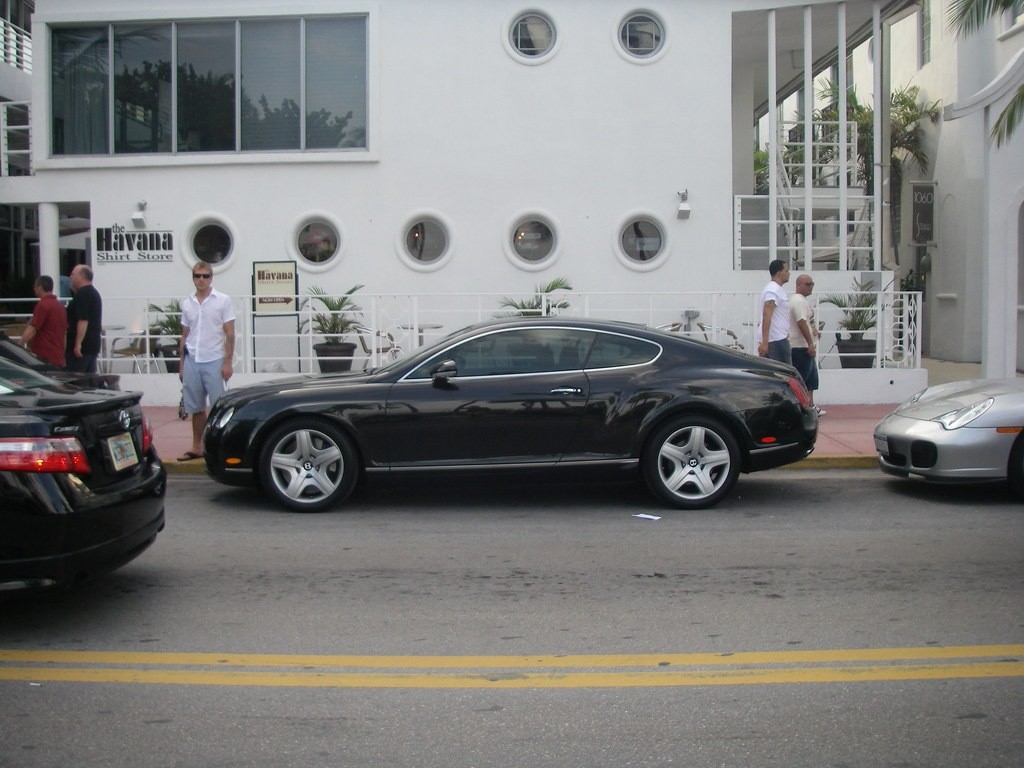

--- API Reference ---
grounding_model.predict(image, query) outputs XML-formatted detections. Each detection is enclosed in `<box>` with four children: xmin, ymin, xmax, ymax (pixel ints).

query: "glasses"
<box><xmin>802</xmin><ymin>281</ymin><xmax>814</xmax><ymax>288</ymax></box>
<box><xmin>193</xmin><ymin>273</ymin><xmax>212</xmax><ymax>279</ymax></box>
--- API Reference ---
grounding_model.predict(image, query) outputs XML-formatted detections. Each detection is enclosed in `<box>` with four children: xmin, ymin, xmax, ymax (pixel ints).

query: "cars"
<box><xmin>201</xmin><ymin>314</ymin><xmax>818</xmax><ymax>512</ymax></box>
<box><xmin>0</xmin><ymin>334</ymin><xmax>166</xmax><ymax>598</ymax></box>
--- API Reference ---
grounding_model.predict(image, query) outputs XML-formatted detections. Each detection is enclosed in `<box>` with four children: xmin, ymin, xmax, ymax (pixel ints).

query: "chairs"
<box><xmin>109</xmin><ymin>328</ymin><xmax>162</xmax><ymax>374</ymax></box>
<box><xmin>353</xmin><ymin>324</ymin><xmax>406</xmax><ymax>369</ymax></box>
<box><xmin>559</xmin><ymin>345</ymin><xmax>580</xmax><ymax>368</ymax></box>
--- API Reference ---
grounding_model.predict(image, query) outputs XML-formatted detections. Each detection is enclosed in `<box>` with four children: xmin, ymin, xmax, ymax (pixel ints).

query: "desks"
<box><xmin>100</xmin><ymin>325</ymin><xmax>126</xmax><ymax>373</ymax></box>
<box><xmin>397</xmin><ymin>322</ymin><xmax>443</xmax><ymax>348</ymax></box>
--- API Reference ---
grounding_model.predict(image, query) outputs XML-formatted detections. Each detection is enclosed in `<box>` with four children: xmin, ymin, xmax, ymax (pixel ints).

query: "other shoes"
<box><xmin>813</xmin><ymin>405</ymin><xmax>827</xmax><ymax>416</ymax></box>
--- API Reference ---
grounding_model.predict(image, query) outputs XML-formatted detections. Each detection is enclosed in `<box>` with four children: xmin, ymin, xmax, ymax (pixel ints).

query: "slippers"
<box><xmin>177</xmin><ymin>450</ymin><xmax>203</xmax><ymax>461</ymax></box>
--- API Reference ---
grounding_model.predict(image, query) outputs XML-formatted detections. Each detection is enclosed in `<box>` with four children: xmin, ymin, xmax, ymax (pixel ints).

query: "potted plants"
<box><xmin>148</xmin><ymin>299</ymin><xmax>183</xmax><ymax>373</ymax></box>
<box><xmin>295</xmin><ymin>283</ymin><xmax>366</xmax><ymax>373</ymax></box>
<box><xmin>819</xmin><ymin>277</ymin><xmax>899</xmax><ymax>368</ymax></box>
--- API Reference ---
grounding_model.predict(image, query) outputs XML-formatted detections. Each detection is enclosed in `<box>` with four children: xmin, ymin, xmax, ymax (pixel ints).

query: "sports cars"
<box><xmin>873</xmin><ymin>373</ymin><xmax>1023</xmax><ymax>503</ymax></box>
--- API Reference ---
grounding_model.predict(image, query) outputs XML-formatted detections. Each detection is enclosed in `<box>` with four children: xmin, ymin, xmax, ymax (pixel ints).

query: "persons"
<box><xmin>788</xmin><ymin>274</ymin><xmax>827</xmax><ymax>417</ymax></box>
<box><xmin>176</xmin><ymin>261</ymin><xmax>235</xmax><ymax>461</ymax></box>
<box><xmin>757</xmin><ymin>261</ymin><xmax>793</xmax><ymax>368</ymax></box>
<box><xmin>66</xmin><ymin>264</ymin><xmax>102</xmax><ymax>373</ymax></box>
<box><xmin>18</xmin><ymin>275</ymin><xmax>66</xmax><ymax>371</ymax></box>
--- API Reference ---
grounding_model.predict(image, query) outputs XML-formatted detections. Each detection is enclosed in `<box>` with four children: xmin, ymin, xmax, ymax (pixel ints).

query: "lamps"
<box><xmin>131</xmin><ymin>201</ymin><xmax>148</xmax><ymax>230</ymax></box>
<box><xmin>677</xmin><ymin>187</ymin><xmax>691</xmax><ymax>220</ymax></box>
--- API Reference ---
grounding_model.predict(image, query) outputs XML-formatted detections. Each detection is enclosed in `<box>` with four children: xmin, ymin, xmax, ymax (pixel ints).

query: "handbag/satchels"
<box><xmin>179</xmin><ymin>380</ymin><xmax>189</xmax><ymax>420</ymax></box>
